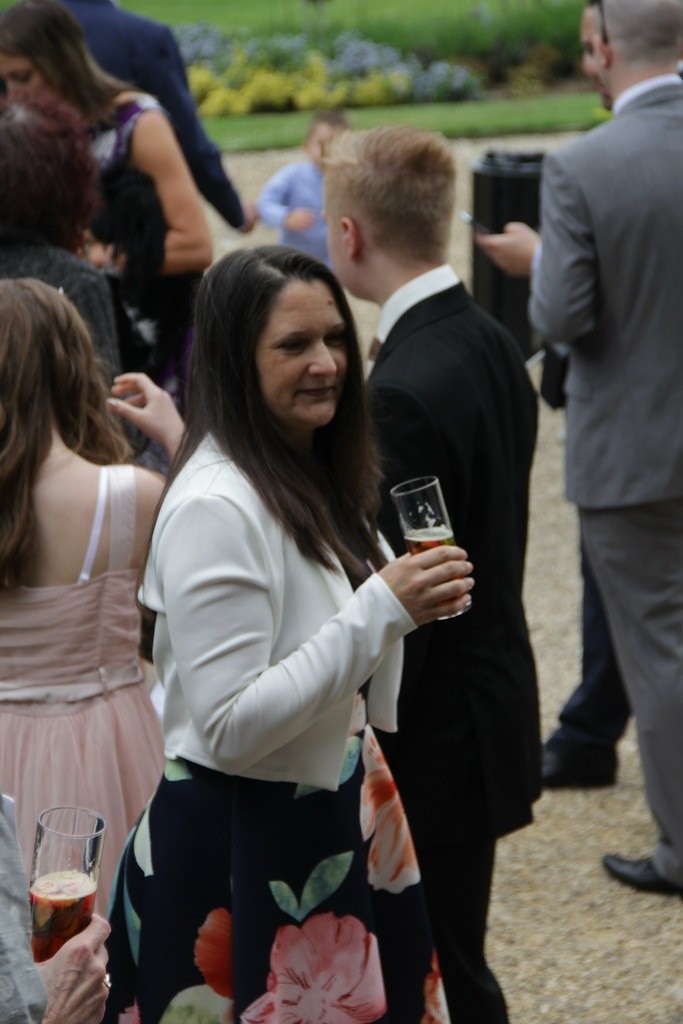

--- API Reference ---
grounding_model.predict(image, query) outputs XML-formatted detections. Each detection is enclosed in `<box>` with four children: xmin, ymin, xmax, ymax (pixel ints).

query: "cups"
<box><xmin>388</xmin><ymin>476</ymin><xmax>473</xmax><ymax>619</ymax></box>
<box><xmin>28</xmin><ymin>806</ymin><xmax>106</xmax><ymax>964</ymax></box>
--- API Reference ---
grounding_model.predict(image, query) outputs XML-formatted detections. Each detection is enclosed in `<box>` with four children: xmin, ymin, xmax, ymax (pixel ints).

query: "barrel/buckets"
<box><xmin>471</xmin><ymin>150</ymin><xmax>547</xmax><ymax>368</ymax></box>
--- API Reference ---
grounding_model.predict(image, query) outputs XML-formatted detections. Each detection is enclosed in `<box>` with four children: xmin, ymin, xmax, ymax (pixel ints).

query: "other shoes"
<box><xmin>540</xmin><ymin>744</ymin><xmax>616</xmax><ymax>786</ymax></box>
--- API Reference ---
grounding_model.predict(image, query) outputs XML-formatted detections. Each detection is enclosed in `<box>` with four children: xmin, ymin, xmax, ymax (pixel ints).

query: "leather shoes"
<box><xmin>602</xmin><ymin>854</ymin><xmax>683</xmax><ymax>902</ymax></box>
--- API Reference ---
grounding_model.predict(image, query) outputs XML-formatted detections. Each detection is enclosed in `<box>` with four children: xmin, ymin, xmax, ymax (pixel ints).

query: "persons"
<box><xmin>0</xmin><ymin>0</ymin><xmax>214</xmax><ymax>419</ymax></box>
<box><xmin>62</xmin><ymin>0</ymin><xmax>259</xmax><ymax>235</ymax></box>
<box><xmin>0</xmin><ymin>794</ymin><xmax>111</xmax><ymax>1024</ymax></box>
<box><xmin>0</xmin><ymin>276</ymin><xmax>191</xmax><ymax>934</ymax></box>
<box><xmin>0</xmin><ymin>94</ymin><xmax>154</xmax><ymax>373</ymax></box>
<box><xmin>259</xmin><ymin>109</ymin><xmax>349</xmax><ymax>272</ymax></box>
<box><xmin>472</xmin><ymin>0</ymin><xmax>682</xmax><ymax>900</ymax></box>
<box><xmin>110</xmin><ymin>245</ymin><xmax>477</xmax><ymax>1024</ymax></box>
<box><xmin>318</xmin><ymin>127</ymin><xmax>544</xmax><ymax>1024</ymax></box>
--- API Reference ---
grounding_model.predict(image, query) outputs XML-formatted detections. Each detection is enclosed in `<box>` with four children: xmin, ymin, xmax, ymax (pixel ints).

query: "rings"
<box><xmin>104</xmin><ymin>975</ymin><xmax>111</xmax><ymax>988</ymax></box>
